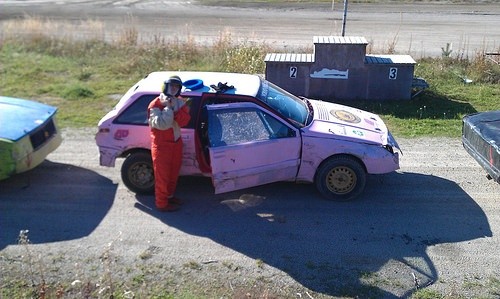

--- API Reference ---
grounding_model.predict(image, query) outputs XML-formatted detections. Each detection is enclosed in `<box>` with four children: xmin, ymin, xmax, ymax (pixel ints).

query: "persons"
<box><xmin>147</xmin><ymin>75</ymin><xmax>191</xmax><ymax>211</ymax></box>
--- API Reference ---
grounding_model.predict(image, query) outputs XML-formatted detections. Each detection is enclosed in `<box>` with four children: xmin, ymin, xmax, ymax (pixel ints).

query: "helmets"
<box><xmin>161</xmin><ymin>75</ymin><xmax>183</xmax><ymax>97</ymax></box>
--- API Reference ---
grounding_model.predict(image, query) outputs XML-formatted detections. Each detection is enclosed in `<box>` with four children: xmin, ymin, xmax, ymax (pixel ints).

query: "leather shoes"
<box><xmin>159</xmin><ymin>204</ymin><xmax>181</xmax><ymax>211</ymax></box>
<box><xmin>168</xmin><ymin>196</ymin><xmax>185</xmax><ymax>204</ymax></box>
